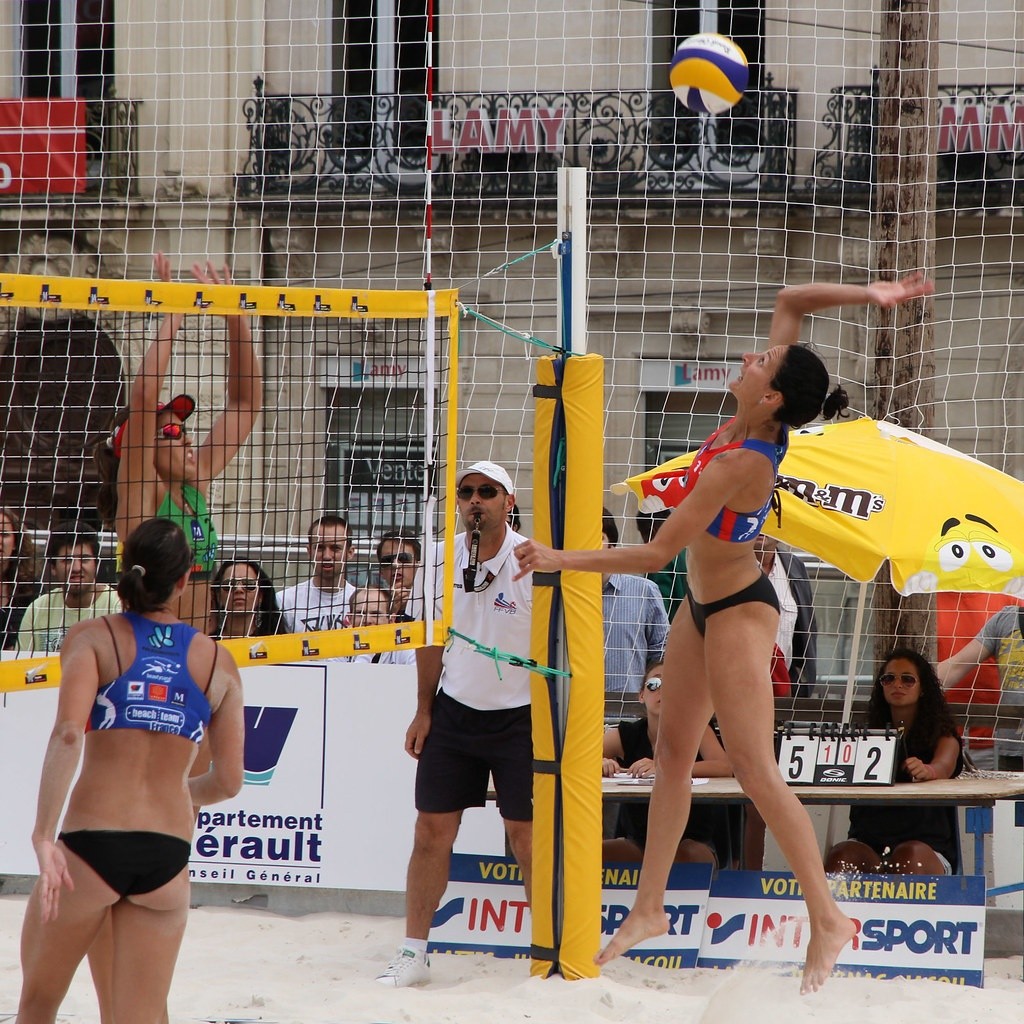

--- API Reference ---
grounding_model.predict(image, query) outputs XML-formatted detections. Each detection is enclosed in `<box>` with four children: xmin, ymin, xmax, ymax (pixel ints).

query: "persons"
<box><xmin>327</xmin><ymin>585</ymin><xmax>417</xmax><ymax>664</ymax></box>
<box><xmin>937</xmin><ymin>604</ymin><xmax>1024</xmax><ymax>826</ymax></box>
<box><xmin>605</xmin><ymin>662</ymin><xmax>730</xmax><ymax>869</ymax></box>
<box><xmin>17</xmin><ymin>522</ymin><xmax>122</xmax><ymax>651</ymax></box>
<box><xmin>602</xmin><ymin>506</ymin><xmax>671</xmax><ymax>692</ymax></box>
<box><xmin>14</xmin><ymin>517</ymin><xmax>225</xmax><ymax>1024</ymax></box>
<box><xmin>0</xmin><ymin>506</ymin><xmax>46</xmax><ymax>650</ymax></box>
<box><xmin>87</xmin><ymin>252</ymin><xmax>263</xmax><ymax>1024</ymax></box>
<box><xmin>375</xmin><ymin>460</ymin><xmax>532</xmax><ymax>988</ymax></box>
<box><xmin>376</xmin><ymin>530</ymin><xmax>421</xmax><ymax>622</ymax></box>
<box><xmin>275</xmin><ymin>515</ymin><xmax>357</xmax><ymax>633</ymax></box>
<box><xmin>754</xmin><ymin>533</ymin><xmax>818</xmax><ymax>698</ymax></box>
<box><xmin>512</xmin><ymin>273</ymin><xmax>935</xmax><ymax>995</ymax></box>
<box><xmin>826</xmin><ymin>649</ymin><xmax>963</xmax><ymax>876</ymax></box>
<box><xmin>206</xmin><ymin>556</ymin><xmax>287</xmax><ymax>642</ymax></box>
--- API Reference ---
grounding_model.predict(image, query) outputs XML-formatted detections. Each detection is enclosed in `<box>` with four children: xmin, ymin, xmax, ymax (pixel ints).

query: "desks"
<box><xmin>601</xmin><ymin>775</ymin><xmax>1024</xmax><ymax>905</ymax></box>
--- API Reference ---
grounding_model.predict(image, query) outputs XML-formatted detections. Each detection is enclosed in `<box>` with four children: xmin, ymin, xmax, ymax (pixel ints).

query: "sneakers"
<box><xmin>375</xmin><ymin>944</ymin><xmax>432</xmax><ymax>988</ymax></box>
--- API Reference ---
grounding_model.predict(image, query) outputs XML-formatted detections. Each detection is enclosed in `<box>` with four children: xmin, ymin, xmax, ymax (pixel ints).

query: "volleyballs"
<box><xmin>670</xmin><ymin>32</ymin><xmax>750</xmax><ymax>114</ymax></box>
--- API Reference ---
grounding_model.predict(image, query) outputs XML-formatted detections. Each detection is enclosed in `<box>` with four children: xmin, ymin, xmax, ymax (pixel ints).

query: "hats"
<box><xmin>456</xmin><ymin>461</ymin><xmax>513</xmax><ymax>495</ymax></box>
<box><xmin>112</xmin><ymin>394</ymin><xmax>195</xmax><ymax>459</ymax></box>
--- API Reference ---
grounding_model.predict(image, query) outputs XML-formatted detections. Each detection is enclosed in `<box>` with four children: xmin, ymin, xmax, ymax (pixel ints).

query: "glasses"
<box><xmin>456</xmin><ymin>484</ymin><xmax>507</xmax><ymax>501</ymax></box>
<box><xmin>878</xmin><ymin>674</ymin><xmax>919</xmax><ymax>689</ymax></box>
<box><xmin>222</xmin><ymin>578</ymin><xmax>256</xmax><ymax>592</ymax></box>
<box><xmin>644</xmin><ymin>677</ymin><xmax>661</xmax><ymax>691</ymax></box>
<box><xmin>381</xmin><ymin>552</ymin><xmax>414</xmax><ymax>568</ymax></box>
<box><xmin>154</xmin><ymin>422</ymin><xmax>187</xmax><ymax>440</ymax></box>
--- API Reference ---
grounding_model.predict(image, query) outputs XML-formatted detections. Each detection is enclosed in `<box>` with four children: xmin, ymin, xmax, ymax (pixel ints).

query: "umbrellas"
<box><xmin>610</xmin><ymin>414</ymin><xmax>1024</xmax><ymax>733</ymax></box>
